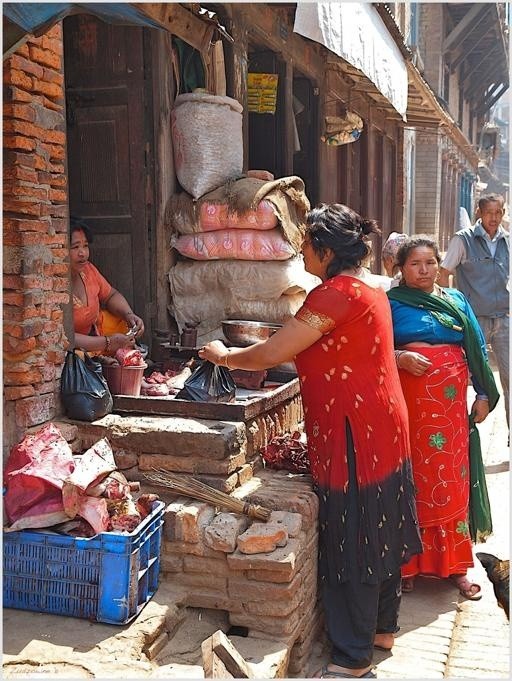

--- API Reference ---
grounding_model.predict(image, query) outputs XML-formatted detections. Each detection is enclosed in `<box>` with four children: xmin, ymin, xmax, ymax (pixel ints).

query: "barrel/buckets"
<box><xmin>321</xmin><ymin>661</ymin><xmax>377</xmax><ymax>679</ymax></box>
<box><xmin>374</xmin><ymin>645</ymin><xmax>392</xmax><ymax>652</ymax></box>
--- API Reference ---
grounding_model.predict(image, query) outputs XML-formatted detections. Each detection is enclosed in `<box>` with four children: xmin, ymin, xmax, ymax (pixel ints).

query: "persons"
<box><xmin>200</xmin><ymin>203</ymin><xmax>423</xmax><ymax>679</ymax></box>
<box><xmin>70</xmin><ymin>220</ymin><xmax>144</xmax><ymax>356</ymax></box>
<box><xmin>382</xmin><ymin>232</ymin><xmax>408</xmax><ymax>289</ymax></box>
<box><xmin>386</xmin><ymin>234</ymin><xmax>500</xmax><ymax>601</ymax></box>
<box><xmin>438</xmin><ymin>193</ymin><xmax>512</xmax><ymax>446</ymax></box>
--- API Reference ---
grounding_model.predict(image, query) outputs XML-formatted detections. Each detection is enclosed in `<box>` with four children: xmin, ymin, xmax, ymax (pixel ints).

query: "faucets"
<box><xmin>401</xmin><ymin>574</ymin><xmax>417</xmax><ymax>593</ymax></box>
<box><xmin>450</xmin><ymin>574</ymin><xmax>483</xmax><ymax>601</ymax></box>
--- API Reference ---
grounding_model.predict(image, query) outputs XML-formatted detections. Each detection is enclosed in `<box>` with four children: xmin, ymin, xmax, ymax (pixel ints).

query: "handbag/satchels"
<box><xmin>104</xmin><ymin>336</ymin><xmax>111</xmax><ymax>354</ymax></box>
<box><xmin>125</xmin><ymin>312</ymin><xmax>133</xmax><ymax>318</ymax></box>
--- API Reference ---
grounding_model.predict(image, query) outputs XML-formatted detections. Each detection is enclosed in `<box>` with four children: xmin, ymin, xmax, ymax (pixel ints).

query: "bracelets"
<box><xmin>219</xmin><ymin>319</ymin><xmax>285</xmax><ymax>346</ymax></box>
<box><xmin>225</xmin><ymin>351</ymin><xmax>236</xmax><ymax>371</ymax></box>
<box><xmin>125</xmin><ymin>312</ymin><xmax>133</xmax><ymax>318</ymax></box>
<box><xmin>396</xmin><ymin>350</ymin><xmax>406</xmax><ymax>368</ymax></box>
<box><xmin>477</xmin><ymin>395</ymin><xmax>489</xmax><ymax>401</ymax></box>
<box><xmin>104</xmin><ymin>336</ymin><xmax>111</xmax><ymax>354</ymax></box>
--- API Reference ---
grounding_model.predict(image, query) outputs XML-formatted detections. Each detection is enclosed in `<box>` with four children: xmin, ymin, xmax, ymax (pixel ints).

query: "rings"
<box><xmin>203</xmin><ymin>346</ymin><xmax>206</xmax><ymax>350</ymax></box>
